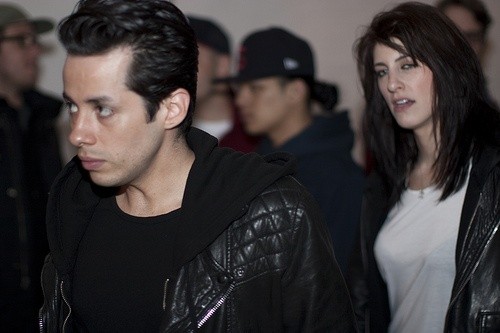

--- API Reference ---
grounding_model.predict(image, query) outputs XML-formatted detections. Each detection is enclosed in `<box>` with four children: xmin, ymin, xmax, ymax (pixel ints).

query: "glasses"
<box><xmin>0</xmin><ymin>35</ymin><xmax>35</xmax><ymax>47</ymax></box>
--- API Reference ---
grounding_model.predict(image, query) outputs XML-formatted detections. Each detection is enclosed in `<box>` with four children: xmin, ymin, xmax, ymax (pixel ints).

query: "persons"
<box><xmin>0</xmin><ymin>0</ymin><xmax>500</xmax><ymax>333</ymax></box>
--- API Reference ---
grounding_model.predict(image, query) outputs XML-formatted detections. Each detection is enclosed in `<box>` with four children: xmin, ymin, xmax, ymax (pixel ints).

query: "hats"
<box><xmin>210</xmin><ymin>27</ymin><xmax>318</xmax><ymax>84</ymax></box>
<box><xmin>0</xmin><ymin>4</ymin><xmax>54</xmax><ymax>34</ymax></box>
